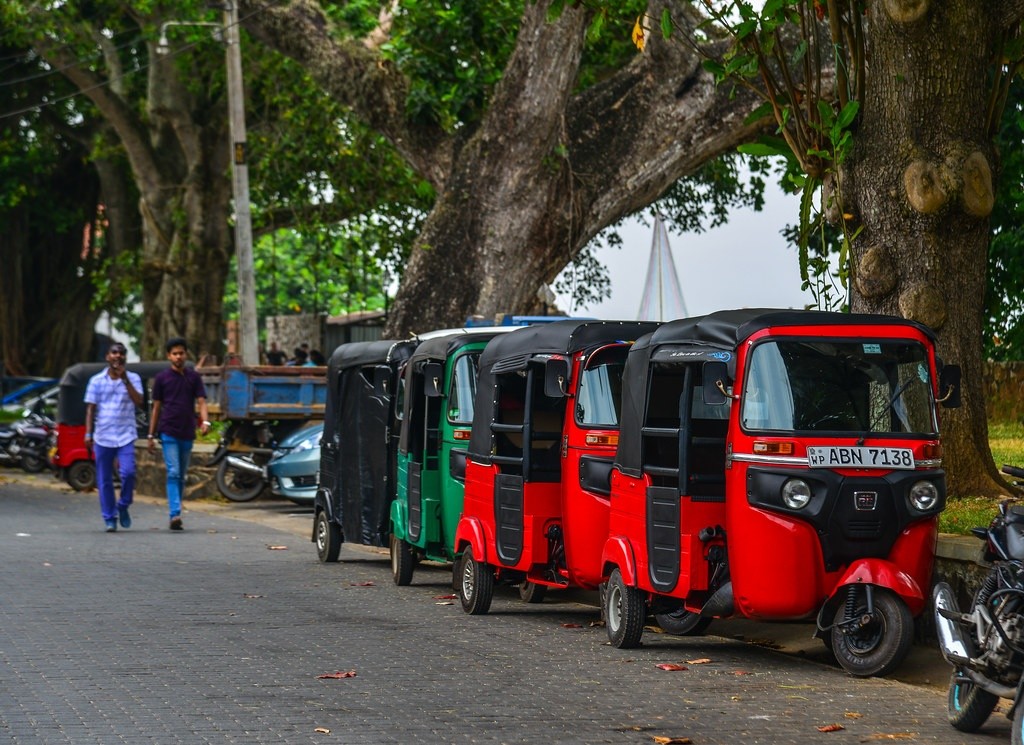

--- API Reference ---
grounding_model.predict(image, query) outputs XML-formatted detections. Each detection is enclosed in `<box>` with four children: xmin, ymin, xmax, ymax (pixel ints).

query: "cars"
<box><xmin>270</xmin><ymin>422</ymin><xmax>322</xmax><ymax>506</ymax></box>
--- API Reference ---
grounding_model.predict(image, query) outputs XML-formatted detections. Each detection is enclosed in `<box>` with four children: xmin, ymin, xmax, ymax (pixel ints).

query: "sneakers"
<box><xmin>119</xmin><ymin>509</ymin><xmax>131</xmax><ymax>528</ymax></box>
<box><xmin>106</xmin><ymin>519</ymin><xmax>116</xmax><ymax>531</ymax></box>
<box><xmin>169</xmin><ymin>517</ymin><xmax>183</xmax><ymax>531</ymax></box>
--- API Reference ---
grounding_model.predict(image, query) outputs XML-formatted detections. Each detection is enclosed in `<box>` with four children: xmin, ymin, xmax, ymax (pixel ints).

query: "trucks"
<box><xmin>190</xmin><ymin>361</ymin><xmax>329</xmax><ymax>427</ymax></box>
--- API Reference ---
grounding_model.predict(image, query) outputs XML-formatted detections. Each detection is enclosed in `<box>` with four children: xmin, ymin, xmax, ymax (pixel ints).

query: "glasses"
<box><xmin>110</xmin><ymin>350</ymin><xmax>125</xmax><ymax>356</ymax></box>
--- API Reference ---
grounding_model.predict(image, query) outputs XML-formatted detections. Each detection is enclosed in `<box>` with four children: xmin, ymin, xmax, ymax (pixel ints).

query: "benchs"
<box><xmin>498</xmin><ymin>425</ymin><xmax>561</xmax><ymax>483</ymax></box>
<box><xmin>647</xmin><ymin>418</ymin><xmax>737</xmax><ymax>496</ymax></box>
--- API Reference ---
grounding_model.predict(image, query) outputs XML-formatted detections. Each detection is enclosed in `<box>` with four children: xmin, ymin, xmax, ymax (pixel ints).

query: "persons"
<box><xmin>147</xmin><ymin>338</ymin><xmax>211</xmax><ymax>531</ymax></box>
<box><xmin>83</xmin><ymin>342</ymin><xmax>145</xmax><ymax>533</ymax></box>
<box><xmin>263</xmin><ymin>341</ymin><xmax>328</xmax><ymax>370</ymax></box>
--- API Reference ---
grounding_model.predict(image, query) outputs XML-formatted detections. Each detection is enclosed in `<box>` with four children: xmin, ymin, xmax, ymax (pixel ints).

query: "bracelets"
<box><xmin>85</xmin><ymin>433</ymin><xmax>91</xmax><ymax>438</ymax></box>
<box><xmin>148</xmin><ymin>435</ymin><xmax>153</xmax><ymax>438</ymax></box>
<box><xmin>122</xmin><ymin>378</ymin><xmax>128</xmax><ymax>385</ymax></box>
<box><xmin>202</xmin><ymin>420</ymin><xmax>210</xmax><ymax>426</ymax></box>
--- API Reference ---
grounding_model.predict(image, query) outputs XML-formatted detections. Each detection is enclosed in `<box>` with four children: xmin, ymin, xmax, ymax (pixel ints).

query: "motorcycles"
<box><xmin>48</xmin><ymin>362</ymin><xmax>178</xmax><ymax>494</ymax></box>
<box><xmin>922</xmin><ymin>461</ymin><xmax>1024</xmax><ymax>745</ymax></box>
<box><xmin>307</xmin><ymin>339</ymin><xmax>427</xmax><ymax>562</ymax></box>
<box><xmin>211</xmin><ymin>428</ymin><xmax>302</xmax><ymax>501</ymax></box>
<box><xmin>387</xmin><ymin>330</ymin><xmax>519</xmax><ymax>586</ymax></box>
<box><xmin>452</xmin><ymin>320</ymin><xmax>662</xmax><ymax>617</ymax></box>
<box><xmin>0</xmin><ymin>400</ymin><xmax>57</xmax><ymax>476</ymax></box>
<box><xmin>592</xmin><ymin>308</ymin><xmax>960</xmax><ymax>677</ymax></box>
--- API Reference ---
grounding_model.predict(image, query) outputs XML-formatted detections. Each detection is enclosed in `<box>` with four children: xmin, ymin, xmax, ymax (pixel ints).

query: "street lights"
<box><xmin>155</xmin><ymin>18</ymin><xmax>260</xmax><ymax>371</ymax></box>
